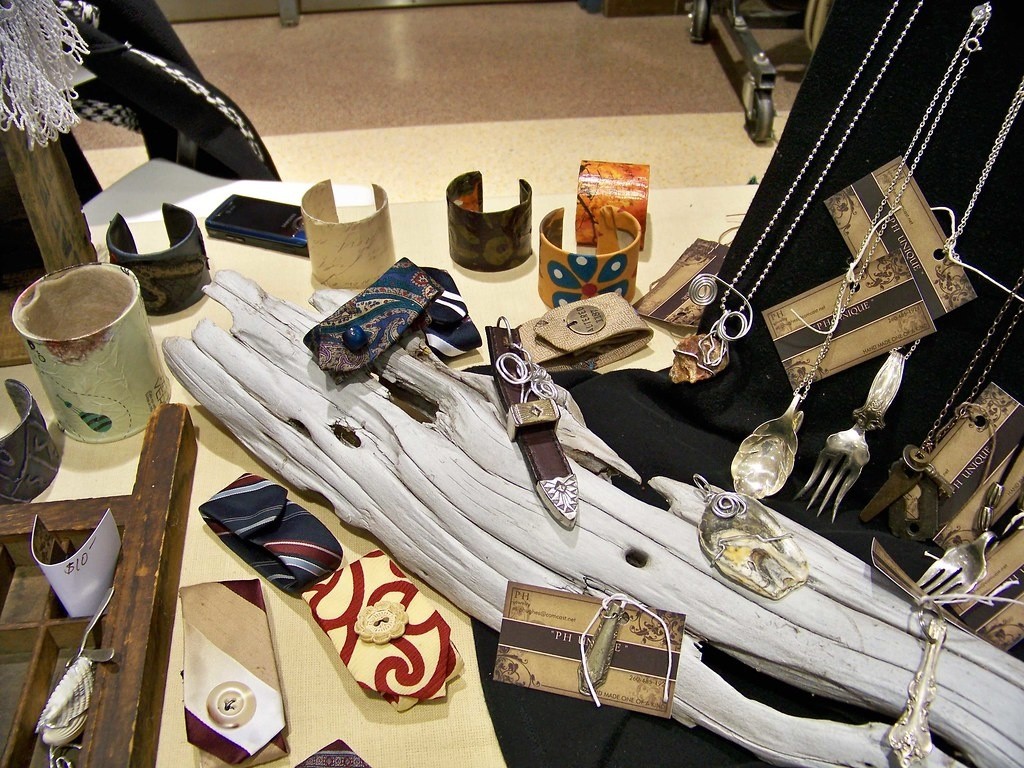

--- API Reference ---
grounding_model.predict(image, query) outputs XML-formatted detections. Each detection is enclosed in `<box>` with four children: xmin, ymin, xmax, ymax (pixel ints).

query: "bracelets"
<box><xmin>0</xmin><ymin>379</ymin><xmax>60</xmax><ymax>503</ymax></box>
<box><xmin>300</xmin><ymin>158</ymin><xmax>650</xmax><ymax>309</ymax></box>
<box><xmin>105</xmin><ymin>203</ymin><xmax>212</xmax><ymax>318</ymax></box>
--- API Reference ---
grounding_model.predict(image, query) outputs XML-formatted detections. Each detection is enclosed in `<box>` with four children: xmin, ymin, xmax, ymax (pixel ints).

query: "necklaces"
<box><xmin>664</xmin><ymin>0</ymin><xmax>1023</xmax><ymax>525</ymax></box>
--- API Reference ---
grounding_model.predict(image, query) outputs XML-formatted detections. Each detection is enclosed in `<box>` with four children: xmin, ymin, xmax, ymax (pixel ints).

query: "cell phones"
<box><xmin>205</xmin><ymin>194</ymin><xmax>312</xmax><ymax>257</ymax></box>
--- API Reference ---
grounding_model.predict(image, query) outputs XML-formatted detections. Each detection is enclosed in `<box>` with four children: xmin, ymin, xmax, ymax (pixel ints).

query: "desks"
<box><xmin>0</xmin><ymin>185</ymin><xmax>759</xmax><ymax>768</ymax></box>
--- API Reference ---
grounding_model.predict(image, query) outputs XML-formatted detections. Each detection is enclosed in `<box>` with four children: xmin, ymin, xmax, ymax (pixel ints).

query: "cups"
<box><xmin>11</xmin><ymin>260</ymin><xmax>177</xmax><ymax>443</ymax></box>
